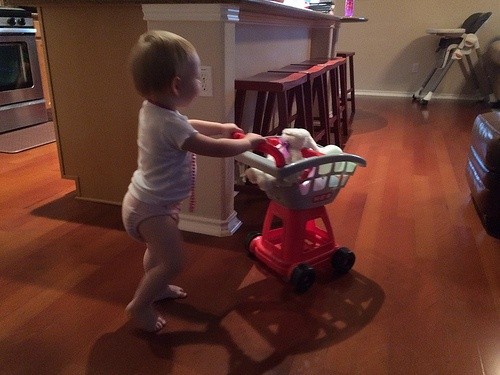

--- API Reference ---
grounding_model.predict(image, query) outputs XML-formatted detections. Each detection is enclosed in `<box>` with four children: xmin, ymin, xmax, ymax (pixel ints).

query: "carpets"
<box><xmin>0</xmin><ymin>121</ymin><xmax>56</xmax><ymax>154</ymax></box>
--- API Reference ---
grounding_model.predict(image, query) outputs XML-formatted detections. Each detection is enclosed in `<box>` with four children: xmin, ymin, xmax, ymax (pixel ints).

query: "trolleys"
<box><xmin>229</xmin><ymin>129</ymin><xmax>370</xmax><ymax>295</ymax></box>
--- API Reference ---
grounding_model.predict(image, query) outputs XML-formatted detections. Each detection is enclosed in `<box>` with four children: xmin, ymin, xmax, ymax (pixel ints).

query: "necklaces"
<box><xmin>189</xmin><ymin>152</ymin><xmax>196</xmax><ymax>212</ymax></box>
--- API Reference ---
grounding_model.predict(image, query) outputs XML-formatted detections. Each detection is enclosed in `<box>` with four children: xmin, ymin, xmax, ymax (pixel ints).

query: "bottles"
<box><xmin>345</xmin><ymin>0</ymin><xmax>355</xmax><ymax>17</ymax></box>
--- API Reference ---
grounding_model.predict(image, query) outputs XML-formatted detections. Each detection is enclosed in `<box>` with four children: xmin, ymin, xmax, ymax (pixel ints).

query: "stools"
<box><xmin>235</xmin><ymin>51</ymin><xmax>356</xmax><ymax>195</ymax></box>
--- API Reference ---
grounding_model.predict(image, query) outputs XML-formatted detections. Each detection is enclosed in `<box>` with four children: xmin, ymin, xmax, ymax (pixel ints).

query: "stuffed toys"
<box><xmin>246</xmin><ymin>127</ymin><xmax>308</xmax><ymax>198</ymax></box>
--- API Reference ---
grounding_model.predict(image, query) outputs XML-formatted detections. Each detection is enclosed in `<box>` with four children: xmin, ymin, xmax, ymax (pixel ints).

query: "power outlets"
<box><xmin>199</xmin><ymin>66</ymin><xmax>213</xmax><ymax>97</ymax></box>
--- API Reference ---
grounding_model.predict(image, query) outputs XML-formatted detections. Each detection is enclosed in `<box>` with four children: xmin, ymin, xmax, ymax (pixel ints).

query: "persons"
<box><xmin>122</xmin><ymin>31</ymin><xmax>268</xmax><ymax>331</ymax></box>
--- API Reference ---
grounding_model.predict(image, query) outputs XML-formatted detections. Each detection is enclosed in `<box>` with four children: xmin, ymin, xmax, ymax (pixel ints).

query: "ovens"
<box><xmin>0</xmin><ymin>26</ymin><xmax>49</xmax><ymax>134</ymax></box>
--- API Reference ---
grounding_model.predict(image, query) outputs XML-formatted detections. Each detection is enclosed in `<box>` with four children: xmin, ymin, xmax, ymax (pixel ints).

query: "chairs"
<box><xmin>413</xmin><ymin>11</ymin><xmax>500</xmax><ymax>108</ymax></box>
